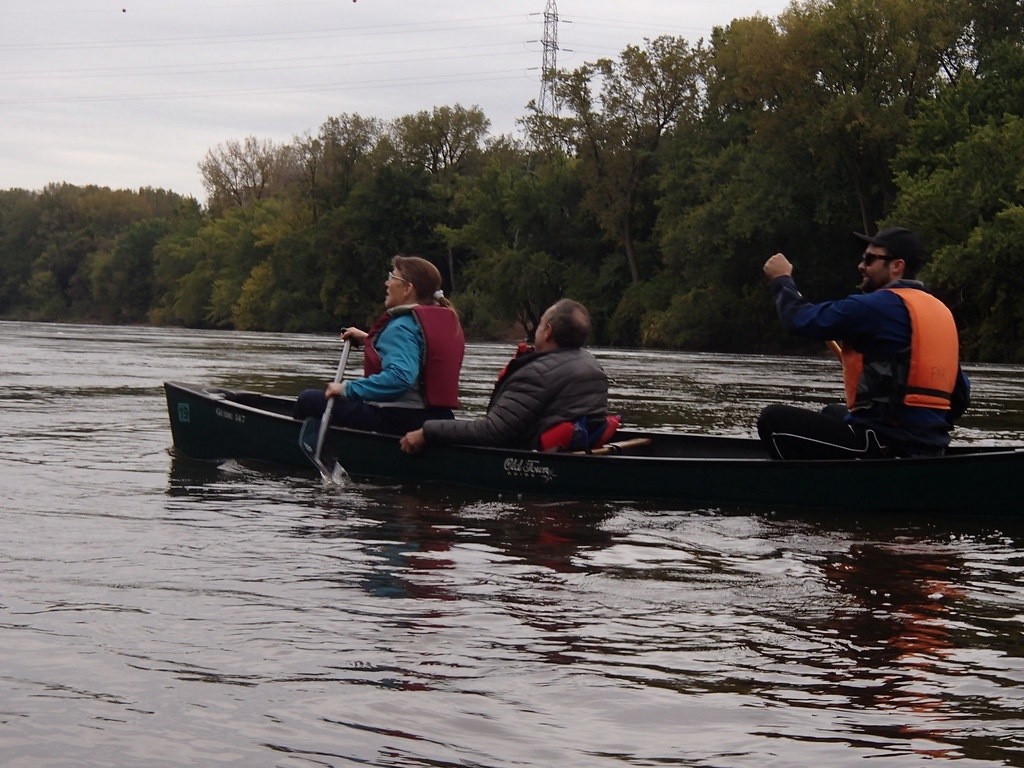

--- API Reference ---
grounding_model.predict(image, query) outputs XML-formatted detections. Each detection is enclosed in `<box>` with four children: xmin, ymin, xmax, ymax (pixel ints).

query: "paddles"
<box><xmin>299</xmin><ymin>327</ymin><xmax>358</xmax><ymax>481</ymax></box>
<box><xmin>794</xmin><ymin>285</ymin><xmax>842</xmax><ymax>360</ymax></box>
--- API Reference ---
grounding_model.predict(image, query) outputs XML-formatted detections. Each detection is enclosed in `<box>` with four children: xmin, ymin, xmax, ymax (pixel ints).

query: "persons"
<box><xmin>400</xmin><ymin>299</ymin><xmax>608</xmax><ymax>453</ymax></box>
<box><xmin>293</xmin><ymin>256</ymin><xmax>465</xmax><ymax>436</ymax></box>
<box><xmin>757</xmin><ymin>227</ymin><xmax>971</xmax><ymax>459</ymax></box>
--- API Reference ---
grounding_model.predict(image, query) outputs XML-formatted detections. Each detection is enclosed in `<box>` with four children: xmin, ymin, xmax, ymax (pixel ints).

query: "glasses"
<box><xmin>863</xmin><ymin>252</ymin><xmax>894</xmax><ymax>266</ymax></box>
<box><xmin>388</xmin><ymin>272</ymin><xmax>407</xmax><ymax>283</ymax></box>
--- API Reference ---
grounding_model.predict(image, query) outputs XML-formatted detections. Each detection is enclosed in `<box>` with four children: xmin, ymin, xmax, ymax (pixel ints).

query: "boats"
<box><xmin>161</xmin><ymin>378</ymin><xmax>1024</xmax><ymax>530</ymax></box>
